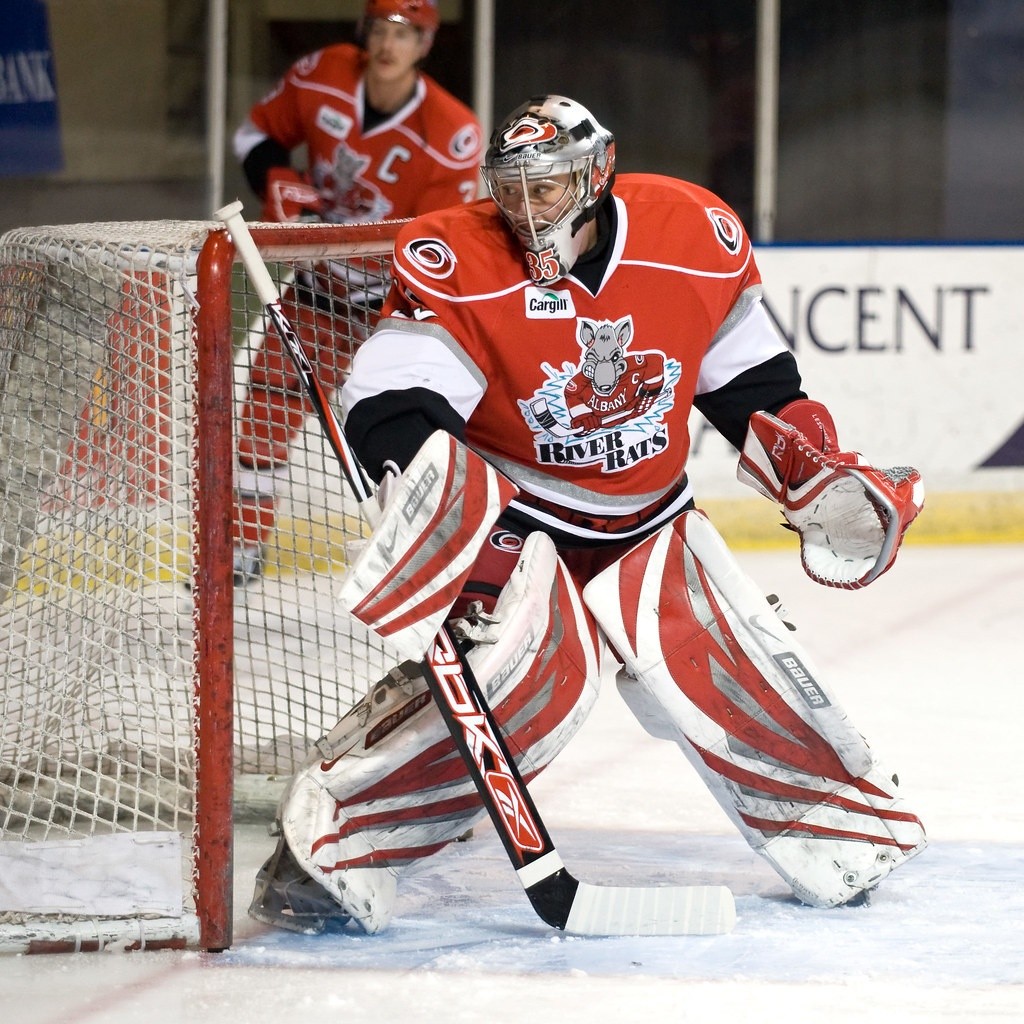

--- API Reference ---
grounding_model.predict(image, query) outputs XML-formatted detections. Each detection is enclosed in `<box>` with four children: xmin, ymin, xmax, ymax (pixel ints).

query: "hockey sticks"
<box><xmin>214</xmin><ymin>190</ymin><xmax>743</xmax><ymax>948</ymax></box>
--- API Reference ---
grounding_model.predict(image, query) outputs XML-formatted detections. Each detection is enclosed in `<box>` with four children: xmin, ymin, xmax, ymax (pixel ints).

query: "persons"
<box><xmin>249</xmin><ymin>93</ymin><xmax>925</xmax><ymax>937</ymax></box>
<box><xmin>184</xmin><ymin>0</ymin><xmax>481</xmax><ymax>592</ymax></box>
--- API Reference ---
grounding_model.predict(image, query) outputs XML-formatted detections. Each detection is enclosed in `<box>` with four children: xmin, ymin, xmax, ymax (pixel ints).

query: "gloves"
<box><xmin>261</xmin><ymin>166</ymin><xmax>326</xmax><ymax>223</ymax></box>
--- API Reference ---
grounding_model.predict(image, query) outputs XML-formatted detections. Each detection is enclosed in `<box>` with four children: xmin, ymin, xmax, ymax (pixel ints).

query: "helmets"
<box><xmin>365</xmin><ymin>0</ymin><xmax>439</xmax><ymax>29</ymax></box>
<box><xmin>478</xmin><ymin>93</ymin><xmax>617</xmax><ymax>287</ymax></box>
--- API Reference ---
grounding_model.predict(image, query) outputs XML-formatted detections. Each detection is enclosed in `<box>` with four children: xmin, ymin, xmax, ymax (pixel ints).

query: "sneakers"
<box><xmin>247</xmin><ymin>813</ymin><xmax>351</xmax><ymax>936</ymax></box>
<box><xmin>184</xmin><ymin>541</ymin><xmax>268</xmax><ymax>603</ymax></box>
